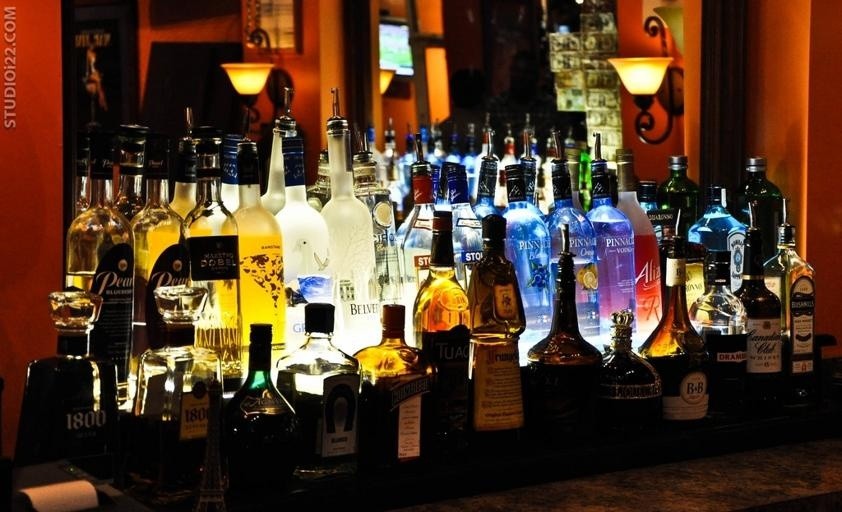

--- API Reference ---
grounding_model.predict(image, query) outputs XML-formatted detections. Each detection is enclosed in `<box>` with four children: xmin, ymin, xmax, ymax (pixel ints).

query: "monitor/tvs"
<box><xmin>144</xmin><ymin>40</ymin><xmax>245</xmax><ymax>133</ymax></box>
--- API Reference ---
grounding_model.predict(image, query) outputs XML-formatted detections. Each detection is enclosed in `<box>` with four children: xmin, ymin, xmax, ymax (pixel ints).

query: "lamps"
<box><xmin>378</xmin><ymin>67</ymin><xmax>395</xmax><ymax>94</ymax></box>
<box><xmin>607</xmin><ymin>11</ymin><xmax>685</xmax><ymax>150</ymax></box>
<box><xmin>213</xmin><ymin>21</ymin><xmax>292</xmax><ymax>135</ymax></box>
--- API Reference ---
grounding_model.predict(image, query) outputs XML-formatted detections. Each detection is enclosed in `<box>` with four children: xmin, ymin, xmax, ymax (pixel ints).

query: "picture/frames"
<box><xmin>244</xmin><ymin>0</ymin><xmax>303</xmax><ymax>55</ymax></box>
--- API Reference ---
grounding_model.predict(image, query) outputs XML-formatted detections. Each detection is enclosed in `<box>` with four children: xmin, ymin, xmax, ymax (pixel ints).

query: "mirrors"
<box><xmin>62</xmin><ymin>0</ymin><xmax>625</xmax><ymax>256</ymax></box>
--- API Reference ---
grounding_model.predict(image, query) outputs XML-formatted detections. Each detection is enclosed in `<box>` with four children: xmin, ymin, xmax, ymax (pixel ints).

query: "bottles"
<box><xmin>14</xmin><ymin>87</ymin><xmax>817</xmax><ymax>511</ymax></box>
